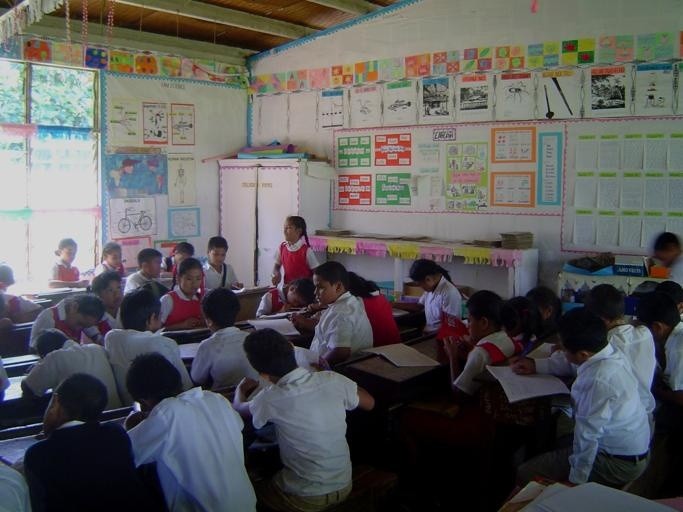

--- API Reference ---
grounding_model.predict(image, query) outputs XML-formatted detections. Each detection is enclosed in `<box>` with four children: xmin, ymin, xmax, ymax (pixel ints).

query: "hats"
<box><xmin>148</xmin><ymin>159</ymin><xmax>160</xmax><ymax>168</ymax></box>
<box><xmin>121</xmin><ymin>159</ymin><xmax>141</xmax><ymax>169</ymax></box>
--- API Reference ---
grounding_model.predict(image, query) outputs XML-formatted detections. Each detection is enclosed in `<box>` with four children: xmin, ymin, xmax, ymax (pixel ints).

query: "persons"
<box><xmin>642</xmin><ymin>81</ymin><xmax>658</xmax><ymax>109</ymax></box>
<box><xmin>502</xmin><ymin>296</ymin><xmax>553</xmax><ymax>359</ymax></box>
<box><xmin>92</xmin><ymin>242</ymin><xmax>126</xmax><ymax>280</ymax></box>
<box><xmin>159</xmin><ymin>257</ymin><xmax>205</xmax><ymax>331</ymax></box>
<box><xmin>202</xmin><ymin>236</ymin><xmax>241</xmax><ymax>289</ymax></box>
<box><xmin>128</xmin><ymin>353</ymin><xmax>258</xmax><ymax>508</ymax></box>
<box><xmin>189</xmin><ymin>288</ymin><xmax>261</xmax><ymax>391</ymax></box>
<box><xmin>104</xmin><ymin>288</ymin><xmax>193</xmax><ymax>407</ymax></box>
<box><xmin>451</xmin><ymin>290</ymin><xmax>520</xmax><ymax>401</ymax></box>
<box><xmin>148</xmin><ymin>113</ymin><xmax>163</xmax><ymax>139</ymax></box>
<box><xmin>344</xmin><ymin>271</ymin><xmax>401</xmax><ymax>346</ymax></box>
<box><xmin>108</xmin><ymin>158</ymin><xmax>140</xmax><ymax>192</ymax></box>
<box><xmin>390</xmin><ymin>258</ymin><xmax>463</xmax><ymax>338</ymax></box>
<box><xmin>272</xmin><ymin>215</ymin><xmax>319</xmax><ymax>306</ymax></box>
<box><xmin>656</xmin><ymin>280</ymin><xmax>683</xmax><ymax>316</ymax></box>
<box><xmin>146</xmin><ymin>158</ymin><xmax>167</xmax><ymax>192</ymax></box>
<box><xmin>173</xmin><ymin>162</ymin><xmax>187</xmax><ymax>207</ymax></box>
<box><xmin>655</xmin><ymin>231</ymin><xmax>682</xmax><ymax>283</ymax></box>
<box><xmin>309</xmin><ymin>261</ymin><xmax>374</xmax><ymax>367</ymax></box>
<box><xmin>83</xmin><ymin>271</ymin><xmax>124</xmax><ymax>344</ymax></box>
<box><xmin>25</xmin><ymin>373</ymin><xmax>138</xmax><ymax>507</ymax></box>
<box><xmin>514</xmin><ymin>308</ymin><xmax>652</xmax><ymax>487</ymax></box>
<box><xmin>49</xmin><ymin>239</ymin><xmax>89</xmax><ymax>288</ymax></box>
<box><xmin>526</xmin><ymin>286</ymin><xmax>563</xmax><ymax>348</ymax></box>
<box><xmin>0</xmin><ymin>265</ymin><xmax>21</xmax><ymax>331</ymax></box>
<box><xmin>122</xmin><ymin>248</ymin><xmax>163</xmax><ymax>294</ymax></box>
<box><xmin>636</xmin><ymin>290</ymin><xmax>683</xmax><ymax>407</ymax></box>
<box><xmin>586</xmin><ymin>283</ymin><xmax>656</xmax><ymax>393</ymax></box>
<box><xmin>232</xmin><ymin>329</ymin><xmax>375</xmax><ymax>508</ymax></box>
<box><xmin>256</xmin><ymin>277</ymin><xmax>315</xmax><ymax>318</ymax></box>
<box><xmin>161</xmin><ymin>243</ymin><xmax>195</xmax><ymax>277</ymax></box>
<box><xmin>21</xmin><ymin>330</ymin><xmax>121</xmax><ymax>416</ymax></box>
<box><xmin>28</xmin><ymin>293</ymin><xmax>104</xmax><ymax>352</ymax></box>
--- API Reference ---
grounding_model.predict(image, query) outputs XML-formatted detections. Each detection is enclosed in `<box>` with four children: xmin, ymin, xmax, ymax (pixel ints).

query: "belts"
<box><xmin>605</xmin><ymin>450</ymin><xmax>649</xmax><ymax>462</ymax></box>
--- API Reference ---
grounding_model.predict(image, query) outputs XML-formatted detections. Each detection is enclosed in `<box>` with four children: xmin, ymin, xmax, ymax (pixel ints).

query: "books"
<box><xmin>484</xmin><ymin>364</ymin><xmax>571</xmax><ymax>403</ymax></box>
<box><xmin>234</xmin><ymin>285</ymin><xmax>272</xmax><ymax>291</ymax></box>
<box><xmin>260</xmin><ymin>308</ymin><xmax>311</xmax><ymax>318</ymax></box>
<box><xmin>502</xmin><ymin>474</ymin><xmax>667</xmax><ymax>508</ymax></box>
<box><xmin>246</xmin><ymin>317</ymin><xmax>299</xmax><ymax>336</ymax></box>
<box><xmin>391</xmin><ymin>306</ymin><xmax>409</xmax><ymax>318</ymax></box>
<box><xmin>358</xmin><ymin>343</ymin><xmax>440</xmax><ymax>368</ymax></box>
